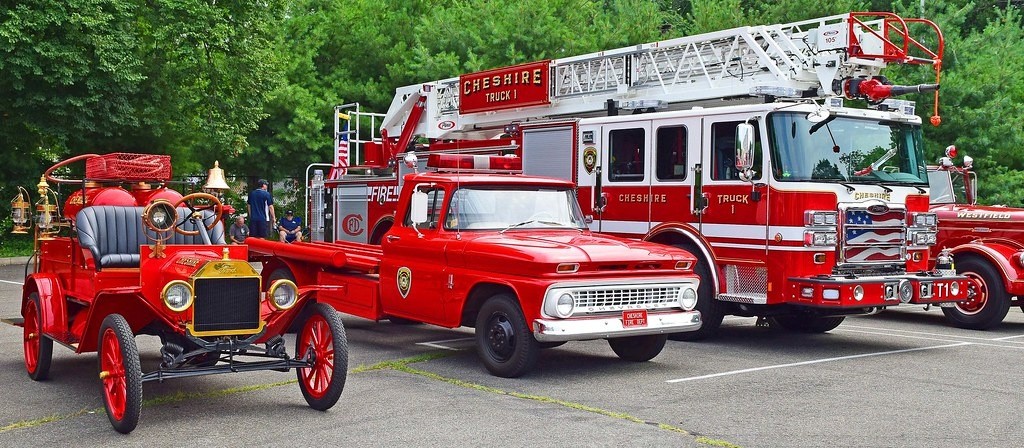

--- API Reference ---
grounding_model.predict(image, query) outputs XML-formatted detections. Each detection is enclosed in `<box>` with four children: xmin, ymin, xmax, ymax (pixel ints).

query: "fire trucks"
<box><xmin>333</xmin><ymin>12</ymin><xmax>969</xmax><ymax>341</ymax></box>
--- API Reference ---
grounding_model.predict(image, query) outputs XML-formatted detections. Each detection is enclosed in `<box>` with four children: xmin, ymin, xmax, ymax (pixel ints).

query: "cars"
<box><xmin>11</xmin><ymin>153</ymin><xmax>348</xmax><ymax>434</ymax></box>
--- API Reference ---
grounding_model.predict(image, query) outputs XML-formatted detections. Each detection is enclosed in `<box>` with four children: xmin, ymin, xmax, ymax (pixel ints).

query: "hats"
<box><xmin>285</xmin><ymin>210</ymin><xmax>293</xmax><ymax>214</ymax></box>
<box><xmin>258</xmin><ymin>179</ymin><xmax>269</xmax><ymax>185</ymax></box>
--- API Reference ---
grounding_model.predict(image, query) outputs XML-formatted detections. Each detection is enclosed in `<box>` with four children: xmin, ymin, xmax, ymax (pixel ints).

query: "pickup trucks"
<box><xmin>245</xmin><ymin>152</ymin><xmax>704</xmax><ymax>378</ymax></box>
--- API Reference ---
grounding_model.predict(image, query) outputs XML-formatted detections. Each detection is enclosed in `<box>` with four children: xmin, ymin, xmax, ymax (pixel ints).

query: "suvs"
<box><xmin>856</xmin><ymin>145</ymin><xmax>1024</xmax><ymax>330</ymax></box>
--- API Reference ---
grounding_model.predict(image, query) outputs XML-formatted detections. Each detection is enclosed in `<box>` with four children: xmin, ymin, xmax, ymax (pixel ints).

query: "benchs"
<box><xmin>76</xmin><ymin>206</ymin><xmax>228</xmax><ymax>273</ymax></box>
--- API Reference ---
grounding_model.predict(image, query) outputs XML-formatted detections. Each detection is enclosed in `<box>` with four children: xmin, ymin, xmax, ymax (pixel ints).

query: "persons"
<box><xmin>278</xmin><ymin>209</ymin><xmax>302</xmax><ymax>243</ymax></box>
<box><xmin>230</xmin><ymin>216</ymin><xmax>249</xmax><ymax>246</ymax></box>
<box><xmin>246</xmin><ymin>179</ymin><xmax>276</xmax><ymax>240</ymax></box>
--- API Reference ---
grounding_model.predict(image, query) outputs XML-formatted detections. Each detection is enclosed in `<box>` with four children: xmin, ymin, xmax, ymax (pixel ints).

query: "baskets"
<box><xmin>85</xmin><ymin>153</ymin><xmax>171</xmax><ymax>180</ymax></box>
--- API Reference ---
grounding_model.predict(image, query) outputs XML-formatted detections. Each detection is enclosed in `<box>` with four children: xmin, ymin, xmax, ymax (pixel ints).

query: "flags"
<box><xmin>329</xmin><ymin>119</ymin><xmax>348</xmax><ymax>181</ymax></box>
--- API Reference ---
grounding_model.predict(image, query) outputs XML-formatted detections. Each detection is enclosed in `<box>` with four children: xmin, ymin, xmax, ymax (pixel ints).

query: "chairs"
<box><xmin>274</xmin><ymin>217</ymin><xmax>310</xmax><ymax>243</ymax></box>
